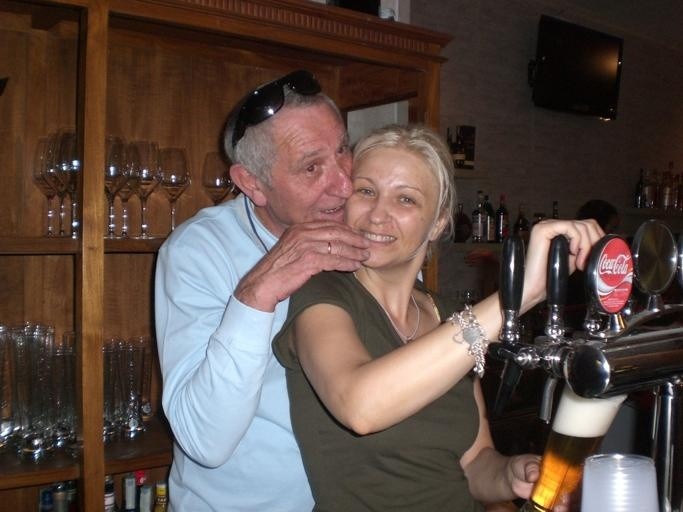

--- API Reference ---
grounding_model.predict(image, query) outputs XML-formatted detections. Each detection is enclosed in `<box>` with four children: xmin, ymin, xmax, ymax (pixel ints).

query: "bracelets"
<box><xmin>449</xmin><ymin>303</ymin><xmax>490</xmax><ymax>378</ymax></box>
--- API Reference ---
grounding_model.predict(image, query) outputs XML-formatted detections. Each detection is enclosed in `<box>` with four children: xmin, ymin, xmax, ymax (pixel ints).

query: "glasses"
<box><xmin>230</xmin><ymin>68</ymin><xmax>322</xmax><ymax>149</ymax></box>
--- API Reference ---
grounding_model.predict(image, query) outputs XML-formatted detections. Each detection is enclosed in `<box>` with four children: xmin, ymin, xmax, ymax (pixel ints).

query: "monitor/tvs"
<box><xmin>528</xmin><ymin>14</ymin><xmax>624</xmax><ymax>120</ymax></box>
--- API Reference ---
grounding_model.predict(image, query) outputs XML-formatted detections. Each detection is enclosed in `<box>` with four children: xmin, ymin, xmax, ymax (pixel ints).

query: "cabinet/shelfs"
<box><xmin>91</xmin><ymin>0</ymin><xmax>455</xmax><ymax>512</ymax></box>
<box><xmin>0</xmin><ymin>1</ymin><xmax>98</xmax><ymax>512</ymax></box>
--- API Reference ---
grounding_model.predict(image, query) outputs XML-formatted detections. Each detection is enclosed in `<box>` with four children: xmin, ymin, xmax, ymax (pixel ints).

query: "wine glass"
<box><xmin>30</xmin><ymin>131</ymin><xmax>190</xmax><ymax>240</ymax></box>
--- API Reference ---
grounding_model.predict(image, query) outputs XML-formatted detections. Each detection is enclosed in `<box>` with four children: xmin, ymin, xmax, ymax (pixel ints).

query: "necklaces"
<box><xmin>353</xmin><ymin>271</ymin><xmax>420</xmax><ymax>341</ymax></box>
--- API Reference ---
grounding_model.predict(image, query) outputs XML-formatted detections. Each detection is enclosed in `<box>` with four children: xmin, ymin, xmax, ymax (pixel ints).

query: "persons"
<box><xmin>270</xmin><ymin>121</ymin><xmax>607</xmax><ymax>511</ymax></box>
<box><xmin>573</xmin><ymin>199</ymin><xmax>619</xmax><ymax>233</ymax></box>
<box><xmin>153</xmin><ymin>68</ymin><xmax>372</xmax><ymax>512</ymax></box>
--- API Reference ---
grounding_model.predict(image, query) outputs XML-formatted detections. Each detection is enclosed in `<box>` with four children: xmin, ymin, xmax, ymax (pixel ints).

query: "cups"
<box><xmin>454</xmin><ymin>289</ymin><xmax>479</xmax><ymax>313</ymax></box>
<box><xmin>0</xmin><ymin>321</ymin><xmax>155</xmax><ymax>467</ymax></box>
<box><xmin>200</xmin><ymin>151</ymin><xmax>235</xmax><ymax>203</ymax></box>
<box><xmin>519</xmin><ymin>390</ymin><xmax>622</xmax><ymax>512</ymax></box>
<box><xmin>579</xmin><ymin>450</ymin><xmax>660</xmax><ymax>512</ymax></box>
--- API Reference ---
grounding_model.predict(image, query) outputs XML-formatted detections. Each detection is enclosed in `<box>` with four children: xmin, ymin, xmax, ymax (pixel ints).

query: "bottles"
<box><xmin>40</xmin><ymin>469</ymin><xmax>168</xmax><ymax>512</ymax></box>
<box><xmin>452</xmin><ymin>190</ymin><xmax>560</xmax><ymax>243</ymax></box>
<box><xmin>450</xmin><ymin>125</ymin><xmax>465</xmax><ymax>167</ymax></box>
<box><xmin>634</xmin><ymin>162</ymin><xmax>683</xmax><ymax>211</ymax></box>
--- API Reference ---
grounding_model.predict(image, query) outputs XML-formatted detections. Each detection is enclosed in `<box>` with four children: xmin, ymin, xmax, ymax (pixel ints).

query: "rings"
<box><xmin>327</xmin><ymin>241</ymin><xmax>331</xmax><ymax>254</ymax></box>
